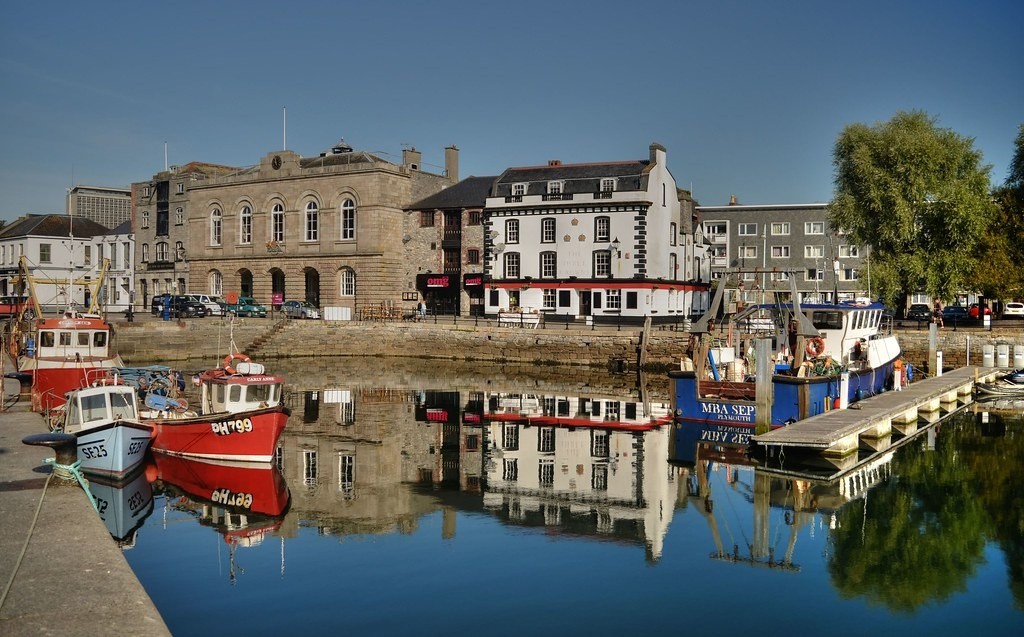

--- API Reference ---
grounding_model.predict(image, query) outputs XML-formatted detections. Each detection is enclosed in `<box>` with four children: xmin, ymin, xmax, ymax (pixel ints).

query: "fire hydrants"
<box><xmin>126</xmin><ymin>305</ymin><xmax>134</xmax><ymax>322</ymax></box>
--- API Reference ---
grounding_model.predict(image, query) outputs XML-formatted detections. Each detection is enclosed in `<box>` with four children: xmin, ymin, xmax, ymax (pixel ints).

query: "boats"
<box><xmin>65</xmin><ymin>359</ymin><xmax>154</xmax><ymax>480</ymax></box>
<box><xmin>667</xmin><ymin>419</ymin><xmax>828</xmax><ymax>574</ymax></box>
<box><xmin>8</xmin><ymin>187</ymin><xmax>128</xmax><ymax>420</ymax></box>
<box><xmin>145</xmin><ymin>450</ymin><xmax>293</xmax><ymax>586</ymax></box>
<box><xmin>974</xmin><ymin>368</ymin><xmax>1024</xmax><ymax>395</ymax></box>
<box><xmin>83</xmin><ymin>474</ymin><xmax>155</xmax><ymax>551</ymax></box>
<box><xmin>134</xmin><ymin>277</ymin><xmax>293</xmax><ymax>462</ymax></box>
<box><xmin>669</xmin><ymin>259</ymin><xmax>902</xmax><ymax>432</ymax></box>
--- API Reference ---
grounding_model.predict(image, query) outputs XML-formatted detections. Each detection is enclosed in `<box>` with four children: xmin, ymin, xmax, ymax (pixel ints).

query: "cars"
<box><xmin>967</xmin><ymin>303</ymin><xmax>991</xmax><ymax>318</ymax></box>
<box><xmin>280</xmin><ymin>300</ymin><xmax>321</xmax><ymax>320</ymax></box>
<box><xmin>1001</xmin><ymin>302</ymin><xmax>1024</xmax><ymax>320</ymax></box>
<box><xmin>941</xmin><ymin>306</ymin><xmax>968</xmax><ymax>321</ymax></box>
<box><xmin>184</xmin><ymin>294</ymin><xmax>227</xmax><ymax>317</ymax></box>
<box><xmin>225</xmin><ymin>296</ymin><xmax>267</xmax><ymax>318</ymax></box>
<box><xmin>907</xmin><ymin>304</ymin><xmax>931</xmax><ymax>320</ymax></box>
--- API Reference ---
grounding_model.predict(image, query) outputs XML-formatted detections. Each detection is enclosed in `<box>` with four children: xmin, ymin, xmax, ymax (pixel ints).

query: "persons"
<box><xmin>932</xmin><ymin>292</ymin><xmax>945</xmax><ymax>329</ymax></box>
<box><xmin>417</xmin><ymin>299</ymin><xmax>427</xmax><ymax>323</ymax></box>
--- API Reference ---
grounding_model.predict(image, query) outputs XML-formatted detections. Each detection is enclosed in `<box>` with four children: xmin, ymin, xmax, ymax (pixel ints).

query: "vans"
<box><xmin>151</xmin><ymin>295</ymin><xmax>206</xmax><ymax>318</ymax></box>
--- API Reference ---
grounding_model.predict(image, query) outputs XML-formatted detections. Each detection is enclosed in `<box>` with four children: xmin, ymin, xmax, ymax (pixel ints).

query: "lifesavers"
<box><xmin>93</xmin><ymin>378</ymin><xmax>125</xmax><ymax>385</ymax></box>
<box><xmin>806</xmin><ymin>337</ymin><xmax>825</xmax><ymax>356</ymax></box>
<box><xmin>223</xmin><ymin>353</ymin><xmax>251</xmax><ymax>375</ymax></box>
